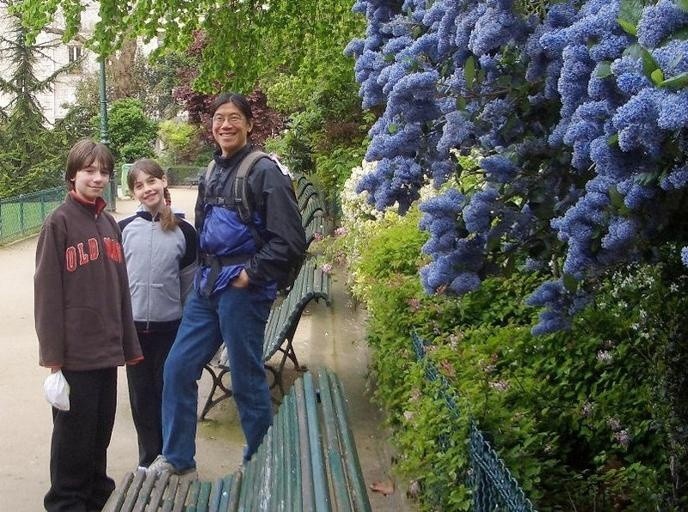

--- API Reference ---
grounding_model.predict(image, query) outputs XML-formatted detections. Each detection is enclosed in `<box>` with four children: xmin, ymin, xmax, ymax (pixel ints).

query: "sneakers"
<box><xmin>145</xmin><ymin>454</ymin><xmax>197</xmax><ymax>477</ymax></box>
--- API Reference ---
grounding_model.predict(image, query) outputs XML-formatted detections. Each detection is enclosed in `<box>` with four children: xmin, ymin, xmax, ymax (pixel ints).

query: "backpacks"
<box><xmin>200</xmin><ymin>153</ymin><xmax>305</xmax><ymax>306</ymax></box>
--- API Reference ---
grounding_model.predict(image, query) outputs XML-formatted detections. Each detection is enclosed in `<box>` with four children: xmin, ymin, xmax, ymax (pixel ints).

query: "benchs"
<box><xmin>99</xmin><ymin>366</ymin><xmax>371</xmax><ymax>512</ymax></box>
<box><xmin>200</xmin><ymin>262</ymin><xmax>331</xmax><ymax>422</ymax></box>
<box><xmin>305</xmin><ymin>216</ymin><xmax>325</xmax><ymax>247</ymax></box>
<box><xmin>297</xmin><ymin>176</ymin><xmax>307</xmax><ymax>183</ymax></box>
<box><xmin>297</xmin><ymin>184</ymin><xmax>318</xmax><ymax>213</ymax></box>
<box><xmin>294</xmin><ymin>178</ymin><xmax>313</xmax><ymax>198</ymax></box>
<box><xmin>302</xmin><ymin>194</ymin><xmax>326</xmax><ymax>225</ymax></box>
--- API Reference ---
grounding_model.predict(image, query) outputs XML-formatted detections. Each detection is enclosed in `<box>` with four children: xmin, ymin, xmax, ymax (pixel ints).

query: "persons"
<box><xmin>151</xmin><ymin>94</ymin><xmax>305</xmax><ymax>482</ymax></box>
<box><xmin>33</xmin><ymin>141</ymin><xmax>144</xmax><ymax>512</ymax></box>
<box><xmin>117</xmin><ymin>159</ymin><xmax>200</xmax><ymax>472</ymax></box>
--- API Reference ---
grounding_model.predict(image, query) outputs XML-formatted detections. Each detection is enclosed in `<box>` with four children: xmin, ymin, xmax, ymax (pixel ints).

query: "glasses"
<box><xmin>212</xmin><ymin>112</ymin><xmax>248</xmax><ymax>123</ymax></box>
